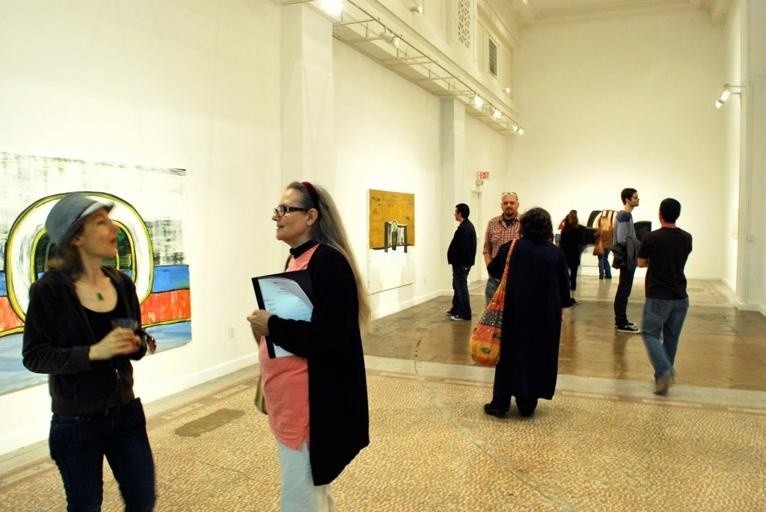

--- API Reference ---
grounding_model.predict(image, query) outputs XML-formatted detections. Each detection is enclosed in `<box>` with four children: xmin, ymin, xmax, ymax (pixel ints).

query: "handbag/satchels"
<box><xmin>593</xmin><ymin>239</ymin><xmax>605</xmax><ymax>256</ymax></box>
<box><xmin>469</xmin><ymin>275</ymin><xmax>509</xmax><ymax>366</ymax></box>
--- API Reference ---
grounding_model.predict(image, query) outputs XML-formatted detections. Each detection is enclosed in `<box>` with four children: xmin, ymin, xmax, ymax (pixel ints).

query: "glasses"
<box><xmin>501</xmin><ymin>192</ymin><xmax>516</xmax><ymax>196</ymax></box>
<box><xmin>272</xmin><ymin>205</ymin><xmax>308</xmax><ymax>217</ymax></box>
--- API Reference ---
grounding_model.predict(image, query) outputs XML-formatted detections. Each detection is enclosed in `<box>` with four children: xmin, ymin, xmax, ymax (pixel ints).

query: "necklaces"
<box><xmin>79</xmin><ymin>279</ymin><xmax>111</xmax><ymax>301</ymax></box>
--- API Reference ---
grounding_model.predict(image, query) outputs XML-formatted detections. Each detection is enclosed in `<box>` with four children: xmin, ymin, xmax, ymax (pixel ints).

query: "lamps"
<box><xmin>714</xmin><ymin>83</ymin><xmax>742</xmax><ymax>108</ymax></box>
<box><xmin>410</xmin><ymin>3</ymin><xmax>424</xmax><ymax>14</ymax></box>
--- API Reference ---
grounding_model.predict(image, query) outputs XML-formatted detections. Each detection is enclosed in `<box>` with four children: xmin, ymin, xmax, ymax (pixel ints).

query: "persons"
<box><xmin>19</xmin><ymin>192</ymin><xmax>159</xmax><ymax>512</ymax></box>
<box><xmin>480</xmin><ymin>206</ymin><xmax>572</xmax><ymax>418</ymax></box>
<box><xmin>244</xmin><ymin>180</ymin><xmax>376</xmax><ymax>512</ymax></box>
<box><xmin>560</xmin><ymin>209</ymin><xmax>594</xmax><ymax>289</ymax></box>
<box><xmin>595</xmin><ymin>216</ymin><xmax>615</xmax><ymax>280</ymax></box>
<box><xmin>611</xmin><ymin>187</ymin><xmax>646</xmax><ymax>335</ymax></box>
<box><xmin>445</xmin><ymin>202</ymin><xmax>477</xmax><ymax>320</ymax></box>
<box><xmin>636</xmin><ymin>196</ymin><xmax>695</xmax><ymax>399</ymax></box>
<box><xmin>483</xmin><ymin>191</ymin><xmax>526</xmax><ymax>306</ymax></box>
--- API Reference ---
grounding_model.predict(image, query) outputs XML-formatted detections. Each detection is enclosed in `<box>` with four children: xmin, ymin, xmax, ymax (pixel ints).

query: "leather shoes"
<box><xmin>483</xmin><ymin>403</ymin><xmax>505</xmax><ymax>417</ymax></box>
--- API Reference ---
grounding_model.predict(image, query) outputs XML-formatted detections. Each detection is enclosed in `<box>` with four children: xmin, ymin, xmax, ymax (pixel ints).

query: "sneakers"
<box><xmin>451</xmin><ymin>315</ymin><xmax>465</xmax><ymax>320</ymax></box>
<box><xmin>618</xmin><ymin>326</ymin><xmax>640</xmax><ymax>333</ymax></box>
<box><xmin>614</xmin><ymin>322</ymin><xmax>636</xmax><ymax>327</ymax></box>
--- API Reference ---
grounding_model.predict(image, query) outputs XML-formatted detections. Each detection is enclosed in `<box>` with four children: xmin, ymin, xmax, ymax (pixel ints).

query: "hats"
<box><xmin>45</xmin><ymin>194</ymin><xmax>114</xmax><ymax>250</ymax></box>
